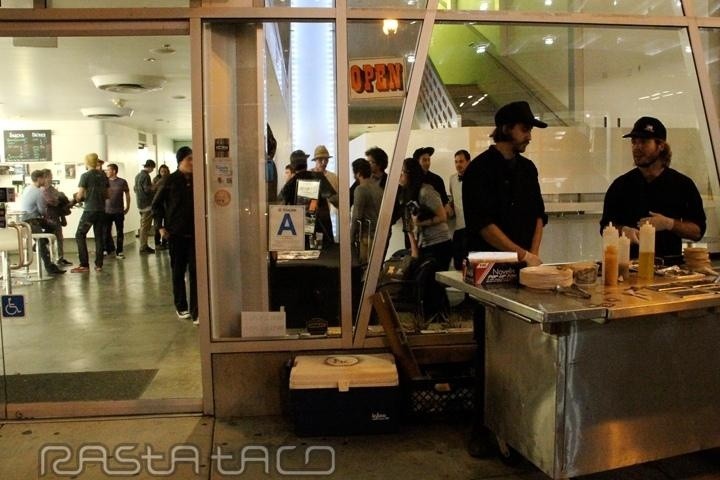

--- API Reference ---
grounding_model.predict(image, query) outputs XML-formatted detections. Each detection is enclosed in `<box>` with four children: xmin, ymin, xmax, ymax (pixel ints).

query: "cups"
<box><xmin>574</xmin><ymin>262</ymin><xmax>600</xmax><ymax>287</ymax></box>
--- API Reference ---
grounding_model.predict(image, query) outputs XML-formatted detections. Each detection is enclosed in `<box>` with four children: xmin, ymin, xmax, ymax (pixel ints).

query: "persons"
<box><xmin>413</xmin><ymin>147</ymin><xmax>452</xmax><ymax>214</ymax></box>
<box><xmin>69</xmin><ymin>153</ymin><xmax>112</xmax><ymax>273</ymax></box>
<box><xmin>103</xmin><ymin>164</ymin><xmax>130</xmax><ymax>259</ymax></box>
<box><xmin>444</xmin><ymin>149</ymin><xmax>472</xmax><ymax>312</ymax></box>
<box><xmin>152</xmin><ymin>146</ymin><xmax>202</xmax><ymax>325</ymax></box>
<box><xmin>134</xmin><ymin>160</ymin><xmax>157</xmax><ymax>254</ymax></box>
<box><xmin>274</xmin><ymin>150</ymin><xmax>338</xmax><ymax>250</ymax></box>
<box><xmin>36</xmin><ymin>170</ymin><xmax>72</xmax><ymax>266</ymax></box>
<box><xmin>461</xmin><ymin>101</ymin><xmax>548</xmax><ymax>459</ymax></box>
<box><xmin>96</xmin><ymin>159</ymin><xmax>110</xmax><ymax>189</ymax></box>
<box><xmin>308</xmin><ymin>146</ymin><xmax>338</xmax><ymax>191</ymax></box>
<box><xmin>283</xmin><ymin>164</ymin><xmax>295</xmax><ymax>182</ymax></box>
<box><xmin>152</xmin><ymin>163</ymin><xmax>170</xmax><ymax>251</ymax></box>
<box><xmin>15</xmin><ymin>169</ymin><xmax>68</xmax><ymax>274</ymax></box>
<box><xmin>600</xmin><ymin>117</ymin><xmax>706</xmax><ymax>267</ymax></box>
<box><xmin>349</xmin><ymin>157</ymin><xmax>383</xmax><ymax>246</ymax></box>
<box><xmin>397</xmin><ymin>157</ymin><xmax>453</xmax><ymax>329</ymax></box>
<box><xmin>350</xmin><ymin>146</ymin><xmax>399</xmax><ymax>269</ymax></box>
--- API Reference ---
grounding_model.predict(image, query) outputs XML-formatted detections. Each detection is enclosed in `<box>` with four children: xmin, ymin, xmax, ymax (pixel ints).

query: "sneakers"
<box><xmin>175</xmin><ymin>309</ymin><xmax>190</xmax><ymax>320</ymax></box>
<box><xmin>192</xmin><ymin>316</ymin><xmax>199</xmax><ymax>325</ymax></box>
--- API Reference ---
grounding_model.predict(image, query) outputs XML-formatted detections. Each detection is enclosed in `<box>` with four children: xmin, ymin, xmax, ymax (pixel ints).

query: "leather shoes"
<box><xmin>139</xmin><ymin>245</ymin><xmax>156</xmax><ymax>254</ymax></box>
<box><xmin>94</xmin><ymin>266</ymin><xmax>103</xmax><ymax>272</ymax></box>
<box><xmin>70</xmin><ymin>265</ymin><xmax>90</xmax><ymax>273</ymax></box>
<box><xmin>48</xmin><ymin>269</ymin><xmax>67</xmax><ymax>276</ymax></box>
<box><xmin>56</xmin><ymin>257</ymin><xmax>73</xmax><ymax>266</ymax></box>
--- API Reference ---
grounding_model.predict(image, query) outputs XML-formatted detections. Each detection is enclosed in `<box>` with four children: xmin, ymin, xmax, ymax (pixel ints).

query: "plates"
<box><xmin>518</xmin><ymin>265</ymin><xmax>575</xmax><ymax>292</ymax></box>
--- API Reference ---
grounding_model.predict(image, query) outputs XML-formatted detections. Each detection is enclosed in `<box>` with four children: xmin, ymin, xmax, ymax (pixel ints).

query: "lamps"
<box><xmin>383</xmin><ymin>19</ymin><xmax>398</xmax><ymax>37</ymax></box>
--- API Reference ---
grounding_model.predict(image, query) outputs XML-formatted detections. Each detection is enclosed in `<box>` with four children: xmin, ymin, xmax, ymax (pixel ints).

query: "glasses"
<box><xmin>369</xmin><ymin>160</ymin><xmax>377</xmax><ymax>165</ymax></box>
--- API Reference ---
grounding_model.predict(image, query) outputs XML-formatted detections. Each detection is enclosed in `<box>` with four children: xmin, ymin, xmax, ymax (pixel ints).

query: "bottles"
<box><xmin>619</xmin><ymin>232</ymin><xmax>629</xmax><ymax>280</ymax></box>
<box><xmin>602</xmin><ymin>221</ymin><xmax>618</xmax><ymax>286</ymax></box>
<box><xmin>639</xmin><ymin>220</ymin><xmax>655</xmax><ymax>283</ymax></box>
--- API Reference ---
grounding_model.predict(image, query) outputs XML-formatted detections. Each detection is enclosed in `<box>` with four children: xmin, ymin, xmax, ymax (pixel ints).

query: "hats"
<box><xmin>141</xmin><ymin>159</ymin><xmax>156</xmax><ymax>168</ymax></box>
<box><xmin>413</xmin><ymin>146</ymin><xmax>435</xmax><ymax>160</ymax></box>
<box><xmin>494</xmin><ymin>100</ymin><xmax>548</xmax><ymax>129</ymax></box>
<box><xmin>311</xmin><ymin>145</ymin><xmax>333</xmax><ymax>161</ymax></box>
<box><xmin>83</xmin><ymin>153</ymin><xmax>99</xmax><ymax>168</ymax></box>
<box><xmin>176</xmin><ymin>146</ymin><xmax>192</xmax><ymax>164</ymax></box>
<box><xmin>290</xmin><ymin>150</ymin><xmax>310</xmax><ymax>163</ymax></box>
<box><xmin>622</xmin><ymin>116</ymin><xmax>667</xmax><ymax>142</ymax></box>
